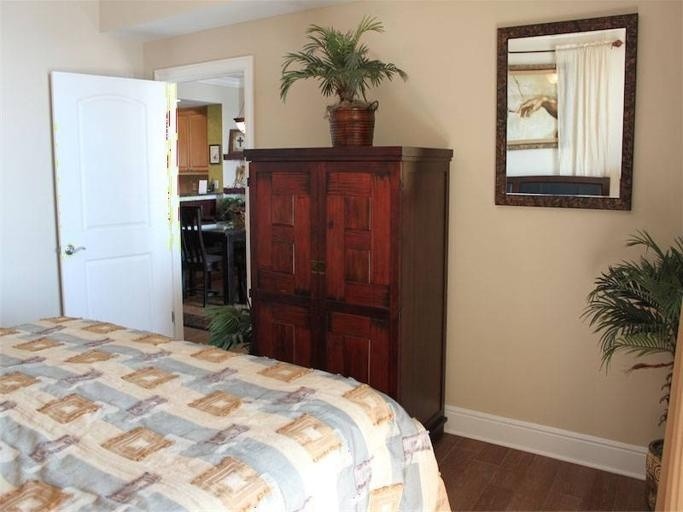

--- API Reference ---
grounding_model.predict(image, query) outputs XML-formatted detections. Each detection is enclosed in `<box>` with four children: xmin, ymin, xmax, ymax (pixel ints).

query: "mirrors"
<box><xmin>495</xmin><ymin>12</ymin><xmax>638</xmax><ymax>211</ymax></box>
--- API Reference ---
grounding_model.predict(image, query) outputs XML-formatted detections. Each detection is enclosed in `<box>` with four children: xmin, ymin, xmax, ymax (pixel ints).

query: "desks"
<box><xmin>182</xmin><ymin>219</ymin><xmax>246</xmax><ymax>304</ymax></box>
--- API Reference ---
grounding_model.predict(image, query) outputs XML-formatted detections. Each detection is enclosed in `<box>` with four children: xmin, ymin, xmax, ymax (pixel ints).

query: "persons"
<box><xmin>516</xmin><ymin>95</ymin><xmax>557</xmax><ymax>119</ymax></box>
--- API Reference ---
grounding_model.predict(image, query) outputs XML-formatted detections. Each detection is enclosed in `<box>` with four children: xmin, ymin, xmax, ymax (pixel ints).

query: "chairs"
<box><xmin>181</xmin><ymin>205</ymin><xmax>223</xmax><ymax>309</ymax></box>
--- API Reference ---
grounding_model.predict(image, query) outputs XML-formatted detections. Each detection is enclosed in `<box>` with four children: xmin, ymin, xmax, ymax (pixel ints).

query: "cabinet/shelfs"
<box><xmin>178</xmin><ymin>105</ymin><xmax>210</xmax><ymax>177</ymax></box>
<box><xmin>243</xmin><ymin>147</ymin><xmax>454</xmax><ymax>445</ymax></box>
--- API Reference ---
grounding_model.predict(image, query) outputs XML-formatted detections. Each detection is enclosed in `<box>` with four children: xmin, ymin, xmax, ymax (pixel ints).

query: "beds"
<box><xmin>2</xmin><ymin>316</ymin><xmax>445</xmax><ymax>510</ymax></box>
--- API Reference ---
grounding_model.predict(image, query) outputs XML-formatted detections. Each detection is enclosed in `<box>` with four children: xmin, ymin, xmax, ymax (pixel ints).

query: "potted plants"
<box><xmin>280</xmin><ymin>14</ymin><xmax>408</xmax><ymax>147</ymax></box>
<box><xmin>581</xmin><ymin>227</ymin><xmax>683</xmax><ymax>512</ymax></box>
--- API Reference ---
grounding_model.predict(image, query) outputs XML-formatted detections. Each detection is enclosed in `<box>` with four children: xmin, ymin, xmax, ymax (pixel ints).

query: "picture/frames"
<box><xmin>506</xmin><ymin>64</ymin><xmax>557</xmax><ymax>152</ymax></box>
<box><xmin>228</xmin><ymin>129</ymin><xmax>245</xmax><ymax>153</ymax></box>
<box><xmin>208</xmin><ymin>145</ymin><xmax>222</xmax><ymax>165</ymax></box>
